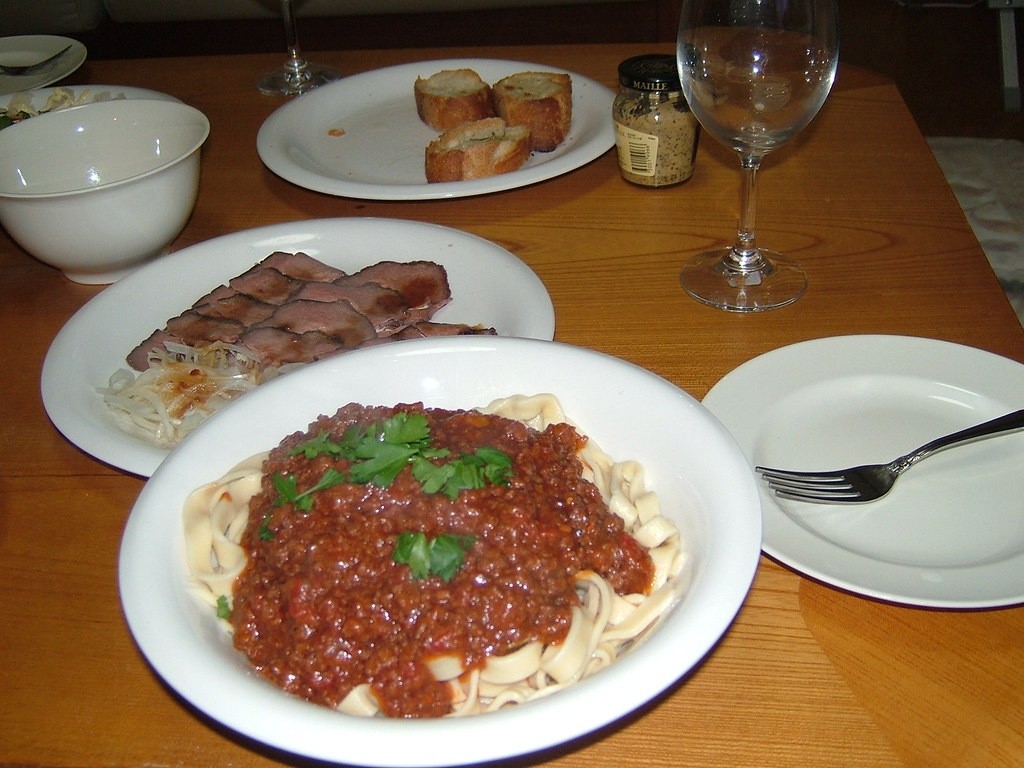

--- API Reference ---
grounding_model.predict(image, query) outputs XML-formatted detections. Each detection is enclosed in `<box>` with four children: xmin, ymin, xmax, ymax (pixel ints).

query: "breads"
<box><xmin>491</xmin><ymin>70</ymin><xmax>573</xmax><ymax>153</ymax></box>
<box><xmin>424</xmin><ymin>115</ymin><xmax>531</xmax><ymax>183</ymax></box>
<box><xmin>414</xmin><ymin>68</ymin><xmax>495</xmax><ymax>132</ymax></box>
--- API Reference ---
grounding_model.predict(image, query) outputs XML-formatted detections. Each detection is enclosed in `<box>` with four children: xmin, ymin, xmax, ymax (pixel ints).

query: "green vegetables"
<box><xmin>216</xmin><ymin>409</ymin><xmax>513</xmax><ymax>622</ymax></box>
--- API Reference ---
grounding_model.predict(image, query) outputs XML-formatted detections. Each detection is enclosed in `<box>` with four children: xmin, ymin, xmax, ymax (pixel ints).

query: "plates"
<box><xmin>41</xmin><ymin>216</ymin><xmax>554</xmax><ymax>479</ymax></box>
<box><xmin>256</xmin><ymin>58</ymin><xmax>616</xmax><ymax>201</ymax></box>
<box><xmin>700</xmin><ymin>334</ymin><xmax>1024</xmax><ymax>608</ymax></box>
<box><xmin>119</xmin><ymin>334</ymin><xmax>763</xmax><ymax>768</ymax></box>
<box><xmin>0</xmin><ymin>85</ymin><xmax>189</xmax><ymax>111</ymax></box>
<box><xmin>0</xmin><ymin>35</ymin><xmax>87</xmax><ymax>97</ymax></box>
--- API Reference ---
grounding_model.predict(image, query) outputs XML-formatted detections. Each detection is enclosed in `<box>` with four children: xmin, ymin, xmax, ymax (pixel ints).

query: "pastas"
<box><xmin>180</xmin><ymin>393</ymin><xmax>689</xmax><ymax>721</ymax></box>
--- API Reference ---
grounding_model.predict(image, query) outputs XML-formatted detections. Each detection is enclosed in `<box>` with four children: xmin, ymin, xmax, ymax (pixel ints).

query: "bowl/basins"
<box><xmin>0</xmin><ymin>99</ymin><xmax>210</xmax><ymax>285</ymax></box>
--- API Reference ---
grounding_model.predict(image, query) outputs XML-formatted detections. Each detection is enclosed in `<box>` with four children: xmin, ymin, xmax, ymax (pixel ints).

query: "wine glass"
<box><xmin>255</xmin><ymin>0</ymin><xmax>341</xmax><ymax>96</ymax></box>
<box><xmin>676</xmin><ymin>0</ymin><xmax>840</xmax><ymax>314</ymax></box>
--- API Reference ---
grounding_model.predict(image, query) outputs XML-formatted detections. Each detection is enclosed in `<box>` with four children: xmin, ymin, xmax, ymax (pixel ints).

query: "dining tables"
<box><xmin>1</xmin><ymin>42</ymin><xmax>1024</xmax><ymax>768</ymax></box>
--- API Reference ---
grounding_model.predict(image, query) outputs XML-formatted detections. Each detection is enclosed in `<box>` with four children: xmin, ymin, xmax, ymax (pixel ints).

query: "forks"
<box><xmin>0</xmin><ymin>44</ymin><xmax>72</xmax><ymax>74</ymax></box>
<box><xmin>755</xmin><ymin>408</ymin><xmax>1024</xmax><ymax>504</ymax></box>
